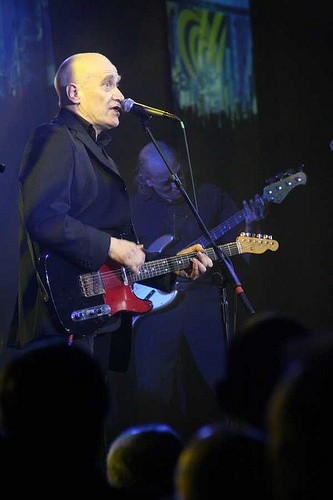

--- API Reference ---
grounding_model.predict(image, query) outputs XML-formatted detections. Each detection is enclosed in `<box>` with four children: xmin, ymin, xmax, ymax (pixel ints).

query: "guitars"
<box><xmin>130</xmin><ymin>167</ymin><xmax>306</xmax><ymax>328</ymax></box>
<box><xmin>43</xmin><ymin>230</ymin><xmax>280</xmax><ymax>340</ymax></box>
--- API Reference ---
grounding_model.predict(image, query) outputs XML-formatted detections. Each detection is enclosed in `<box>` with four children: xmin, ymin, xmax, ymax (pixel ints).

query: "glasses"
<box><xmin>144</xmin><ymin>163</ymin><xmax>183</xmax><ymax>191</ymax></box>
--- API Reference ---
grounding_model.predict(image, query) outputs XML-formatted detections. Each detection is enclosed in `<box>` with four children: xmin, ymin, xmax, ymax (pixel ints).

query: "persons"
<box><xmin>125</xmin><ymin>139</ymin><xmax>268</xmax><ymax>435</ymax></box>
<box><xmin>14</xmin><ymin>51</ymin><xmax>215</xmax><ymax>406</ymax></box>
<box><xmin>0</xmin><ymin>312</ymin><xmax>332</xmax><ymax>499</ymax></box>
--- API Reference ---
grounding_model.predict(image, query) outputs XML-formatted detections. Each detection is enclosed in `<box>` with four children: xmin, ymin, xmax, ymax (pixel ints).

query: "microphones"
<box><xmin>120</xmin><ymin>98</ymin><xmax>182</xmax><ymax>123</ymax></box>
